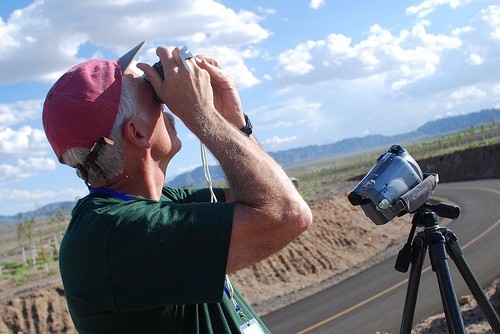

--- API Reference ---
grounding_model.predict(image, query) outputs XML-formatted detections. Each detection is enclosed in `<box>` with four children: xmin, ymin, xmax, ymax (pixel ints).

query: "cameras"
<box><xmin>348</xmin><ymin>144</ymin><xmax>440</xmax><ymax>228</ymax></box>
<box><xmin>140</xmin><ymin>45</ymin><xmax>196</xmax><ymax>104</ymax></box>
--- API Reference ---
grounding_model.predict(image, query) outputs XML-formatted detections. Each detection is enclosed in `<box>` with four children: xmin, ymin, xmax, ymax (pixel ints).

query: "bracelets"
<box><xmin>239</xmin><ymin>111</ymin><xmax>253</xmax><ymax>136</ymax></box>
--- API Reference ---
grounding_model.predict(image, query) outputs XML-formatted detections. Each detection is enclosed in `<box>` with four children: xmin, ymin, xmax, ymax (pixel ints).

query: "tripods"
<box><xmin>394</xmin><ymin>211</ymin><xmax>500</xmax><ymax>334</ymax></box>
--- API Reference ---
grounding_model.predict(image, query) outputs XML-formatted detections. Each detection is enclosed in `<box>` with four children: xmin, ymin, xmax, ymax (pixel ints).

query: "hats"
<box><xmin>43</xmin><ymin>40</ymin><xmax>146</xmax><ymax>190</ymax></box>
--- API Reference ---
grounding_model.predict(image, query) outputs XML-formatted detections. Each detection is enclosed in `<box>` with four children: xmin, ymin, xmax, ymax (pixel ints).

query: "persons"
<box><xmin>41</xmin><ymin>40</ymin><xmax>313</xmax><ymax>334</ymax></box>
